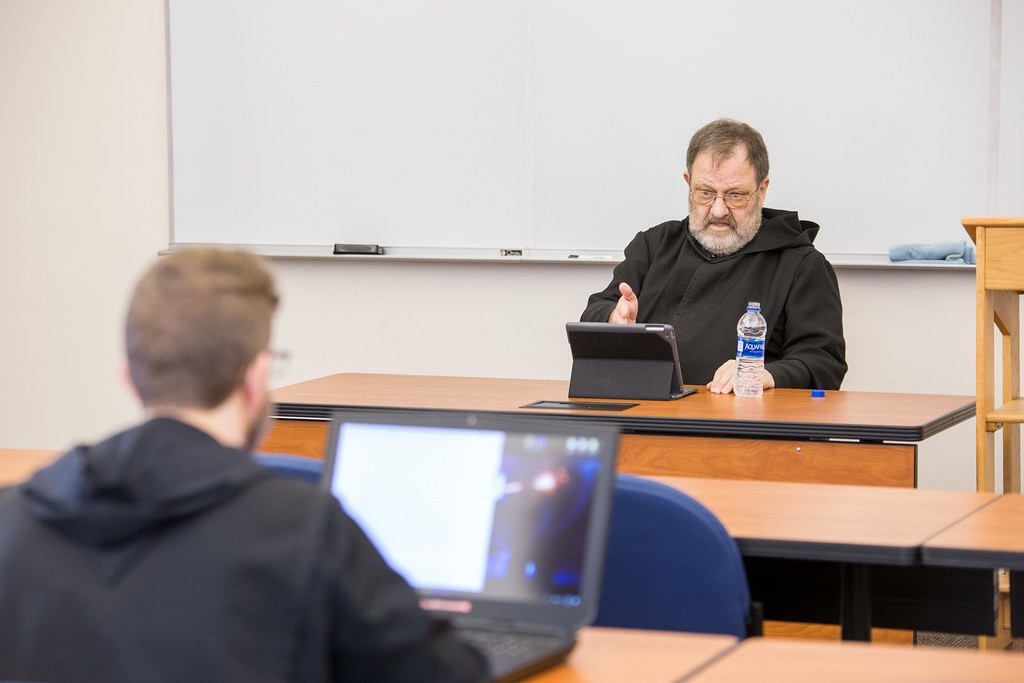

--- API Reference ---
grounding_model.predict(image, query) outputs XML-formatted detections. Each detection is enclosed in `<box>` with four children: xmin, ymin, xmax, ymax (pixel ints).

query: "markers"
<box><xmin>568</xmin><ymin>254</ymin><xmax>614</xmax><ymax>260</ymax></box>
<box><xmin>500</xmin><ymin>248</ymin><xmax>523</xmax><ymax>257</ymax></box>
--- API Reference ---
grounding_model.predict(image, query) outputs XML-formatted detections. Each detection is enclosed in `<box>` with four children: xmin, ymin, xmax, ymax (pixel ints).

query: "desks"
<box><xmin>0</xmin><ymin>365</ymin><xmax>1024</xmax><ymax>683</ymax></box>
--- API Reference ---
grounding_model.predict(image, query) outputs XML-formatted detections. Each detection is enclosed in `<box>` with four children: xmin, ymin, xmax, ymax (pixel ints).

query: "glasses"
<box><xmin>261</xmin><ymin>347</ymin><xmax>292</xmax><ymax>382</ymax></box>
<box><xmin>689</xmin><ymin>176</ymin><xmax>759</xmax><ymax>210</ymax></box>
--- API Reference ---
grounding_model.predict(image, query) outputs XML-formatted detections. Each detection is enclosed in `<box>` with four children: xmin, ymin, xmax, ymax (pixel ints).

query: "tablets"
<box><xmin>566</xmin><ymin>321</ymin><xmax>685</xmax><ymax>393</ymax></box>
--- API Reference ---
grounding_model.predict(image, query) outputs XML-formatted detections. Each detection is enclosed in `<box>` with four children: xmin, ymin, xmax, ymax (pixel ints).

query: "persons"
<box><xmin>579</xmin><ymin>118</ymin><xmax>848</xmax><ymax>395</ymax></box>
<box><xmin>0</xmin><ymin>243</ymin><xmax>491</xmax><ymax>683</ymax></box>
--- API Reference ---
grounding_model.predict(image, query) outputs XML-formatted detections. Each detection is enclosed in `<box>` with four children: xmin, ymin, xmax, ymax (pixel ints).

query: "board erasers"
<box><xmin>334</xmin><ymin>243</ymin><xmax>385</xmax><ymax>256</ymax></box>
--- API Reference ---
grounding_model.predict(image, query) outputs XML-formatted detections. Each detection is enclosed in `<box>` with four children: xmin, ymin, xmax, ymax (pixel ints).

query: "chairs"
<box><xmin>586</xmin><ymin>472</ymin><xmax>764</xmax><ymax>638</ymax></box>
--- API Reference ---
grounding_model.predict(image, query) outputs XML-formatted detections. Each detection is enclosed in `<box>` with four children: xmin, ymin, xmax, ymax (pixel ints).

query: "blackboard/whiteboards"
<box><xmin>157</xmin><ymin>0</ymin><xmax>1007</xmax><ymax>273</ymax></box>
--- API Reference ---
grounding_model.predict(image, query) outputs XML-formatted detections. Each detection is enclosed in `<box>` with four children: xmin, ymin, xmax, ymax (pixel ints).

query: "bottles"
<box><xmin>733</xmin><ymin>301</ymin><xmax>768</xmax><ymax>398</ymax></box>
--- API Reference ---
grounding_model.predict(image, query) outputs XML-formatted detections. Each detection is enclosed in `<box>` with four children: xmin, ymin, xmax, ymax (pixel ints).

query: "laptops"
<box><xmin>323</xmin><ymin>407</ymin><xmax>622</xmax><ymax>683</ymax></box>
